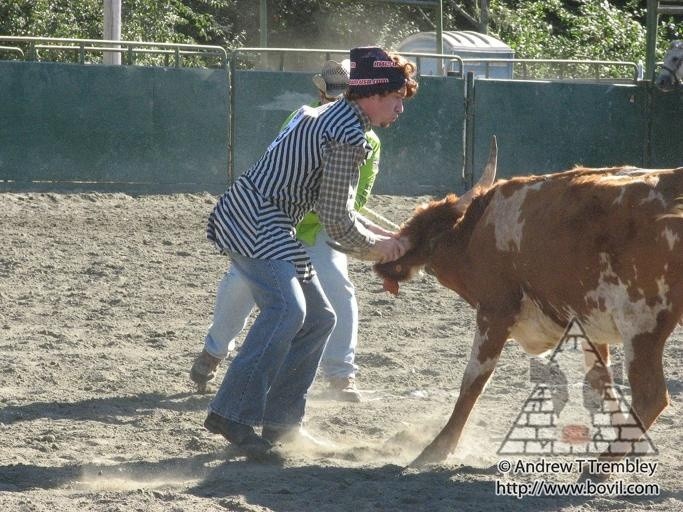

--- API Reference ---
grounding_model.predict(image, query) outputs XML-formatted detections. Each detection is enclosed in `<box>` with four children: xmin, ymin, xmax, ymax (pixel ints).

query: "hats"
<box><xmin>312</xmin><ymin>59</ymin><xmax>352</xmax><ymax>100</ymax></box>
<box><xmin>346</xmin><ymin>46</ymin><xmax>406</xmax><ymax>88</ymax></box>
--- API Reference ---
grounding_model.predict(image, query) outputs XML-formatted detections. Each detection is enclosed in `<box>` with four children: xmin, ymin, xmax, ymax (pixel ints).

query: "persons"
<box><xmin>191</xmin><ymin>47</ymin><xmax>418</xmax><ymax>455</ymax></box>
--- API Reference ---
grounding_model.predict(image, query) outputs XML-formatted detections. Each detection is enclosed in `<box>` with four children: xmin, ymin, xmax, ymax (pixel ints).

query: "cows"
<box><xmin>324</xmin><ymin>134</ymin><xmax>683</xmax><ymax>477</ymax></box>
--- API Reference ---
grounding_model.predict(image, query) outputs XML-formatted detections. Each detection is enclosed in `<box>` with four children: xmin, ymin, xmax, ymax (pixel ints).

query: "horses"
<box><xmin>655</xmin><ymin>40</ymin><xmax>683</xmax><ymax>93</ymax></box>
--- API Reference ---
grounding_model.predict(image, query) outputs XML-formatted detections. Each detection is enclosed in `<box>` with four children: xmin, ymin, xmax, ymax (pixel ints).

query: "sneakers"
<box><xmin>190</xmin><ymin>349</ymin><xmax>225</xmax><ymax>383</ymax></box>
<box><xmin>262</xmin><ymin>426</ymin><xmax>328</xmax><ymax>448</ymax></box>
<box><xmin>329</xmin><ymin>373</ymin><xmax>360</xmax><ymax>404</ymax></box>
<box><xmin>204</xmin><ymin>409</ymin><xmax>255</xmax><ymax>448</ymax></box>
